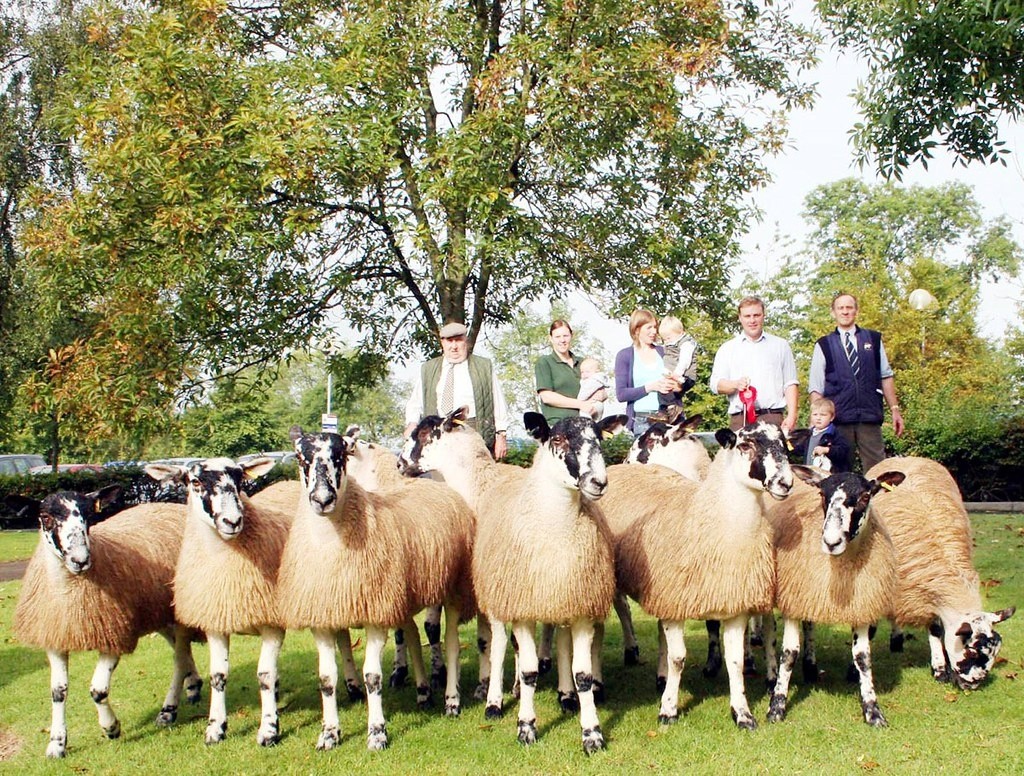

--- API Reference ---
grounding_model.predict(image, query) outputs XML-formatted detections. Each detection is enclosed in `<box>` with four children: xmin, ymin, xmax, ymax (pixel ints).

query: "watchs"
<box><xmin>496</xmin><ymin>432</ymin><xmax>507</xmax><ymax>437</ymax></box>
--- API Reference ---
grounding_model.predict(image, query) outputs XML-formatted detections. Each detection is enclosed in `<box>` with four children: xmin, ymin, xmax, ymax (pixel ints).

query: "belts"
<box><xmin>732</xmin><ymin>408</ymin><xmax>783</xmax><ymax>416</ymax></box>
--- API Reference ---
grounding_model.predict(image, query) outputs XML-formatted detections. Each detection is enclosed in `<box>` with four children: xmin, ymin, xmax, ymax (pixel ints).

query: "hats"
<box><xmin>439</xmin><ymin>322</ymin><xmax>467</xmax><ymax>339</ymax></box>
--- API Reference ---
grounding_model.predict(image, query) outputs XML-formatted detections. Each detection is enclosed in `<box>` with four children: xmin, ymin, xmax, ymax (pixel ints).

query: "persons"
<box><xmin>405</xmin><ymin>324</ymin><xmax>508</xmax><ymax>462</ymax></box>
<box><xmin>636</xmin><ymin>317</ymin><xmax>697</xmax><ymax>426</ymax></box>
<box><xmin>808</xmin><ymin>294</ymin><xmax>903</xmax><ymax>476</ymax></box>
<box><xmin>783</xmin><ymin>398</ymin><xmax>853</xmax><ymax>476</ymax></box>
<box><xmin>535</xmin><ymin>320</ymin><xmax>608</xmax><ymax>426</ymax></box>
<box><xmin>710</xmin><ymin>296</ymin><xmax>800</xmax><ymax>433</ymax></box>
<box><xmin>615</xmin><ymin>306</ymin><xmax>684</xmax><ymax>438</ymax></box>
<box><xmin>577</xmin><ymin>358</ymin><xmax>609</xmax><ymax>422</ymax></box>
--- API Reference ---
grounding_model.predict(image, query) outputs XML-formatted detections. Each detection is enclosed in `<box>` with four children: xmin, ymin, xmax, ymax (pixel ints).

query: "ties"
<box><xmin>441</xmin><ymin>362</ymin><xmax>454</xmax><ymax>415</ymax></box>
<box><xmin>845</xmin><ymin>332</ymin><xmax>860</xmax><ymax>379</ymax></box>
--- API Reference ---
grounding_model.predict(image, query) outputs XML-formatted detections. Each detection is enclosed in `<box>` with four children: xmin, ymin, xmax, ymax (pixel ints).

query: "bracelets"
<box><xmin>889</xmin><ymin>404</ymin><xmax>899</xmax><ymax>411</ymax></box>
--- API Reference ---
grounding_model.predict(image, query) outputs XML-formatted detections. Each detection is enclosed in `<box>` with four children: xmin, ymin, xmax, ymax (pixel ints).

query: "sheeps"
<box><xmin>1</xmin><ymin>482</ymin><xmax>208</xmax><ymax>759</ymax></box>
<box><xmin>141</xmin><ymin>456</ymin><xmax>295</xmax><ymax>751</ymax></box>
<box><xmin>275</xmin><ymin>412</ymin><xmax>1018</xmax><ymax>759</ymax></box>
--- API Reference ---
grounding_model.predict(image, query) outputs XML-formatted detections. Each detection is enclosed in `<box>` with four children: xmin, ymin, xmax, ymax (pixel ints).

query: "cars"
<box><xmin>28</xmin><ymin>451</ymin><xmax>295</xmax><ymax>475</ymax></box>
<box><xmin>0</xmin><ymin>453</ymin><xmax>47</xmax><ymax>478</ymax></box>
